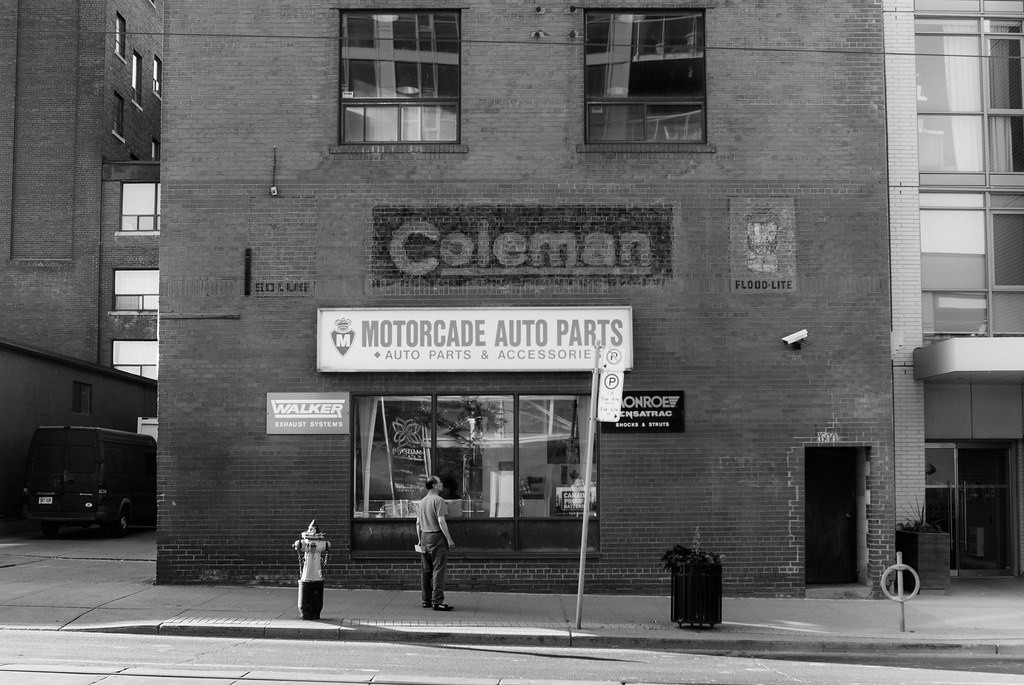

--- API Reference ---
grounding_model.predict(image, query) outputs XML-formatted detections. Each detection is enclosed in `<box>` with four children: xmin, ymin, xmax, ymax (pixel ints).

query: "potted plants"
<box><xmin>895</xmin><ymin>491</ymin><xmax>954</xmax><ymax>597</ymax></box>
<box><xmin>659</xmin><ymin>543</ymin><xmax>726</xmax><ymax>630</ymax></box>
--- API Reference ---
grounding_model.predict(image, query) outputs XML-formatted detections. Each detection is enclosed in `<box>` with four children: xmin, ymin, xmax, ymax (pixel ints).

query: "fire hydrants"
<box><xmin>292</xmin><ymin>518</ymin><xmax>333</xmax><ymax>619</ymax></box>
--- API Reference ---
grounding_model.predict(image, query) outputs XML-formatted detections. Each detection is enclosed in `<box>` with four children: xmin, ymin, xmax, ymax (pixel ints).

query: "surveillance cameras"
<box><xmin>780</xmin><ymin>328</ymin><xmax>808</xmax><ymax>345</ymax></box>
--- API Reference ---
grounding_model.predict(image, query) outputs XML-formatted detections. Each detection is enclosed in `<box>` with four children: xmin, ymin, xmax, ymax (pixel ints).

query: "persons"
<box><xmin>416</xmin><ymin>474</ymin><xmax>455</xmax><ymax>611</ymax></box>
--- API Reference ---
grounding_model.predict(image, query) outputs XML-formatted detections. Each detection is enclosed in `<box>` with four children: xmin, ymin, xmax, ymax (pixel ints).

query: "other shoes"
<box><xmin>434</xmin><ymin>602</ymin><xmax>455</xmax><ymax>611</ymax></box>
<box><xmin>422</xmin><ymin>602</ymin><xmax>433</xmax><ymax>608</ymax></box>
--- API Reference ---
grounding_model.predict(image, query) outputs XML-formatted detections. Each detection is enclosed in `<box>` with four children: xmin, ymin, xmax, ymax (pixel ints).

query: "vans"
<box><xmin>21</xmin><ymin>426</ymin><xmax>158</xmax><ymax>539</ymax></box>
